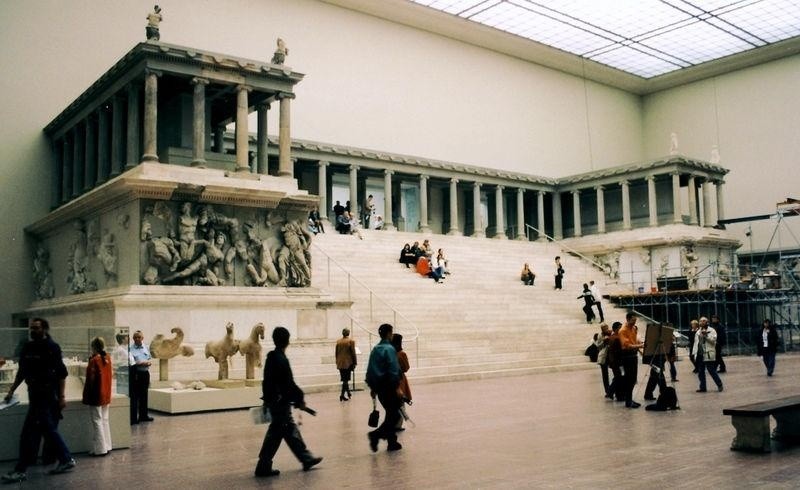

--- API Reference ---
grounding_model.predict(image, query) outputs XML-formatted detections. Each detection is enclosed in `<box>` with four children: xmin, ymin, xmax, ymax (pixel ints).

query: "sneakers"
<box><xmin>2</xmin><ymin>471</ymin><xmax>26</xmax><ymax>482</ymax></box>
<box><xmin>53</xmin><ymin>458</ymin><xmax>77</xmax><ymax>473</ymax></box>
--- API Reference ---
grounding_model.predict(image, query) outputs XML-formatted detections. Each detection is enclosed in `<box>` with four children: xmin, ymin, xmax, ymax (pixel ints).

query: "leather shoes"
<box><xmin>140</xmin><ymin>416</ymin><xmax>154</xmax><ymax>422</ymax></box>
<box><xmin>696</xmin><ymin>389</ymin><xmax>706</xmax><ymax>392</ymax></box>
<box><xmin>626</xmin><ymin>403</ymin><xmax>638</xmax><ymax>408</ymax></box>
<box><xmin>130</xmin><ymin>419</ymin><xmax>139</xmax><ymax>424</ymax></box>
<box><xmin>387</xmin><ymin>444</ymin><xmax>402</xmax><ymax>451</ymax></box>
<box><xmin>368</xmin><ymin>431</ymin><xmax>378</xmax><ymax>452</ymax></box>
<box><xmin>254</xmin><ymin>468</ymin><xmax>280</xmax><ymax>476</ymax></box>
<box><xmin>632</xmin><ymin>401</ymin><xmax>641</xmax><ymax>406</ymax></box>
<box><xmin>718</xmin><ymin>385</ymin><xmax>723</xmax><ymax>392</ymax></box>
<box><xmin>303</xmin><ymin>457</ymin><xmax>323</xmax><ymax>471</ymax></box>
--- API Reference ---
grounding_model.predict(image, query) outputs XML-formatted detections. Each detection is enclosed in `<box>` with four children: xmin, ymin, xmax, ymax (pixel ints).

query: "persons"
<box><xmin>553</xmin><ymin>256</ymin><xmax>564</xmax><ymax>291</ymax></box>
<box><xmin>82</xmin><ymin>337</ymin><xmax>113</xmax><ymax>456</ymax></box>
<box><xmin>375</xmin><ymin>216</ymin><xmax>383</xmax><ymax>230</ymax></box>
<box><xmin>689</xmin><ymin>265</ymin><xmax>700</xmax><ymax>288</ymax></box>
<box><xmin>414</xmin><ymin>253</ymin><xmax>442</xmax><ymax>283</ymax></box>
<box><xmin>335</xmin><ymin>211</ymin><xmax>350</xmax><ymax>234</ymax></box>
<box><xmin>667</xmin><ymin>335</ymin><xmax>679</xmax><ymax>382</ymax></box>
<box><xmin>689</xmin><ymin>320</ymin><xmax>699</xmax><ymax>373</ymax></box>
<box><xmin>400</xmin><ymin>244</ymin><xmax>416</xmax><ymax>268</ymax></box>
<box><xmin>584</xmin><ymin>334</ymin><xmax>599</xmax><ymax>363</ymax></box>
<box><xmin>349</xmin><ymin>212</ymin><xmax>364</xmax><ymax>239</ymax></box>
<box><xmin>266</xmin><ymin>210</ymin><xmax>310</xmax><ymax>287</ymax></box>
<box><xmin>97</xmin><ymin>227</ymin><xmax>117</xmax><ymax>288</ymax></box>
<box><xmin>520</xmin><ymin>263</ymin><xmax>535</xmax><ymax>285</ymax></box>
<box><xmin>709</xmin><ymin>315</ymin><xmax>725</xmax><ymax>373</ymax></box>
<box><xmin>420</xmin><ymin>240</ymin><xmax>434</xmax><ymax>257</ymax></box>
<box><xmin>619</xmin><ymin>312</ymin><xmax>644</xmax><ymax>409</ymax></box>
<box><xmin>365</xmin><ymin>324</ymin><xmax>402</xmax><ymax>453</ymax></box>
<box><xmin>32</xmin><ymin>241</ymin><xmax>54</xmax><ymax>300</ymax></box>
<box><xmin>642</xmin><ymin>354</ymin><xmax>666</xmax><ymax>400</ymax></box>
<box><xmin>336</xmin><ymin>328</ymin><xmax>357</xmax><ymax>401</ymax></box>
<box><xmin>691</xmin><ymin>317</ymin><xmax>723</xmax><ymax>393</ymax></box>
<box><xmin>344</xmin><ymin>201</ymin><xmax>350</xmax><ymax>214</ymax></box>
<box><xmin>588</xmin><ymin>281</ymin><xmax>604</xmax><ymax>324</ymax></box>
<box><xmin>309</xmin><ymin>216</ymin><xmax>317</xmax><ymax>234</ymax></box>
<box><xmin>334</xmin><ymin>201</ymin><xmax>344</xmax><ymax>218</ymax></box>
<box><xmin>178</xmin><ymin>202</ymin><xmax>199</xmax><ymax>285</ymax></box>
<box><xmin>432</xmin><ymin>253</ymin><xmax>445</xmax><ymax>280</ymax></box>
<box><xmin>2</xmin><ymin>318</ymin><xmax>75</xmax><ymax>485</ymax></box>
<box><xmin>129</xmin><ymin>330</ymin><xmax>154</xmax><ymax>425</ymax></box>
<box><xmin>436</xmin><ymin>248</ymin><xmax>450</xmax><ymax>274</ymax></box>
<box><xmin>66</xmin><ymin>219</ymin><xmax>97</xmax><ymax>296</ymax></box>
<box><xmin>391</xmin><ymin>334</ymin><xmax>411</xmax><ymax>431</ymax></box>
<box><xmin>163</xmin><ymin>231</ymin><xmax>226</xmax><ymax>286</ymax></box>
<box><xmin>605</xmin><ymin>321</ymin><xmax>624</xmax><ymax>401</ymax></box>
<box><xmin>416</xmin><ymin>221</ymin><xmax>421</xmax><ymax>232</ymax></box>
<box><xmin>576</xmin><ymin>283</ymin><xmax>596</xmax><ymax>324</ymax></box>
<box><xmin>310</xmin><ymin>204</ymin><xmax>324</xmax><ymax>232</ymax></box>
<box><xmin>365</xmin><ymin>195</ymin><xmax>375</xmax><ymax>229</ymax></box>
<box><xmin>112</xmin><ymin>329</ymin><xmax>136</xmax><ymax>426</ymax></box>
<box><xmin>30</xmin><ymin>333</ymin><xmax>64</xmax><ymax>467</ymax></box>
<box><xmin>598</xmin><ymin>322</ymin><xmax>611</xmax><ymax>398</ymax></box>
<box><xmin>255</xmin><ymin>327</ymin><xmax>324</xmax><ymax>476</ymax></box>
<box><xmin>756</xmin><ymin>320</ymin><xmax>778</xmax><ymax>375</ymax></box>
<box><xmin>410</xmin><ymin>242</ymin><xmax>426</xmax><ymax>260</ymax></box>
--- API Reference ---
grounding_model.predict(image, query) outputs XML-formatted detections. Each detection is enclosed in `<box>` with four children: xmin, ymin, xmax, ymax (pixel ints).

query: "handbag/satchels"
<box><xmin>368</xmin><ymin>396</ymin><xmax>379</xmax><ymax>427</ymax></box>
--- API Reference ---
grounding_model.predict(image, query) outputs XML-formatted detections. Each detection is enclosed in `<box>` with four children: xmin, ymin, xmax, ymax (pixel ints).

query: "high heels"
<box><xmin>340</xmin><ymin>396</ymin><xmax>348</xmax><ymax>401</ymax></box>
<box><xmin>348</xmin><ymin>394</ymin><xmax>352</xmax><ymax>399</ymax></box>
